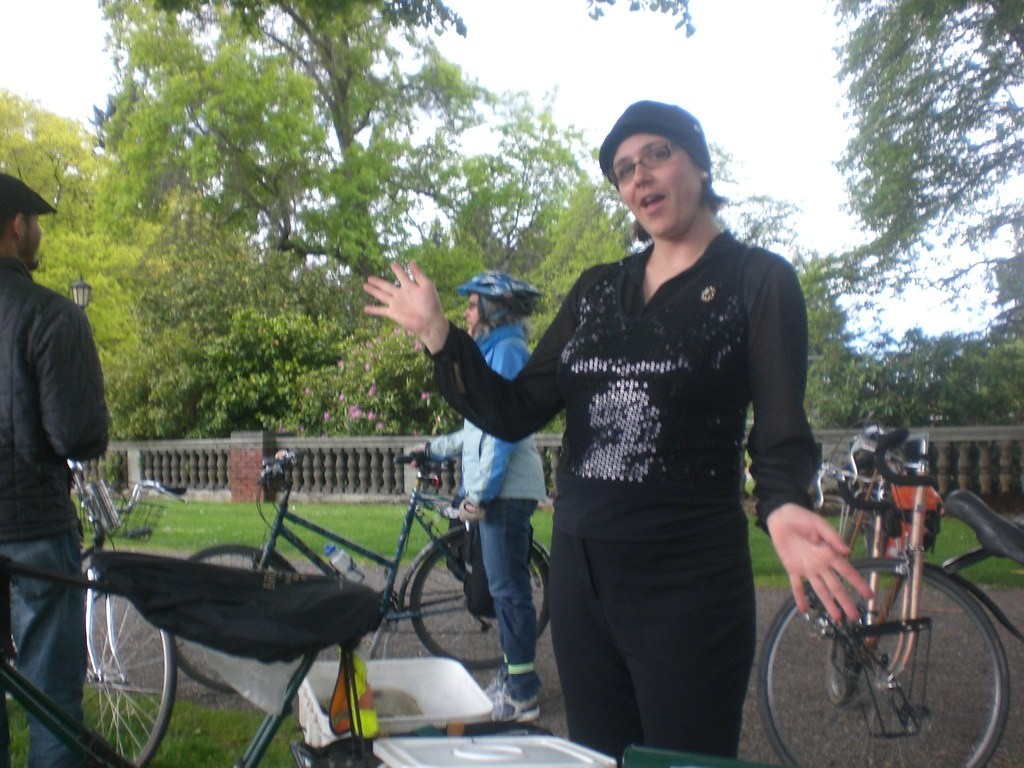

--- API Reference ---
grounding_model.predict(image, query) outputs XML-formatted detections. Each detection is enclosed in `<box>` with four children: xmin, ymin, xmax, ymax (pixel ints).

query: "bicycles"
<box><xmin>0</xmin><ymin>540</ymin><xmax>558</xmax><ymax>768</ymax></box>
<box><xmin>59</xmin><ymin>458</ymin><xmax>187</xmax><ymax>768</ymax></box>
<box><xmin>166</xmin><ymin>439</ymin><xmax>553</xmax><ymax>695</ymax></box>
<box><xmin>752</xmin><ymin>413</ymin><xmax>1014</xmax><ymax>768</ymax></box>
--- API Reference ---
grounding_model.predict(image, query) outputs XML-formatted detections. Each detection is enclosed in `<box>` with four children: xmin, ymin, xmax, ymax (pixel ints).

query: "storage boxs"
<box><xmin>373</xmin><ymin>735</ymin><xmax>619</xmax><ymax>768</ymax></box>
<box><xmin>297</xmin><ymin>658</ymin><xmax>493</xmax><ymax>749</ymax></box>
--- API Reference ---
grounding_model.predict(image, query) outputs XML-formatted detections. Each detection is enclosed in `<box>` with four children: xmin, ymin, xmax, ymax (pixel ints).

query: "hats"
<box><xmin>0</xmin><ymin>174</ymin><xmax>60</xmax><ymax>216</ymax></box>
<box><xmin>599</xmin><ymin>100</ymin><xmax>712</xmax><ymax>188</ymax></box>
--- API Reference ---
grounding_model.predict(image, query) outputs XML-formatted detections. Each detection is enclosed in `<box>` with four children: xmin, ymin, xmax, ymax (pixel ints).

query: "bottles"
<box><xmin>325</xmin><ymin>544</ymin><xmax>366</xmax><ymax>584</ymax></box>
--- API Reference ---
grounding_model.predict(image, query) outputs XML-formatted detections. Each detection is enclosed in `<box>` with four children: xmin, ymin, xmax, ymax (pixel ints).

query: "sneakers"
<box><xmin>484</xmin><ymin>675</ymin><xmax>541</xmax><ymax>722</ymax></box>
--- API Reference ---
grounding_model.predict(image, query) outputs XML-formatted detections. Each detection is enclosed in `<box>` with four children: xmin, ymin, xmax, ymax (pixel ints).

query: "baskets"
<box><xmin>76</xmin><ymin>479</ymin><xmax>164</xmax><ymax>538</ymax></box>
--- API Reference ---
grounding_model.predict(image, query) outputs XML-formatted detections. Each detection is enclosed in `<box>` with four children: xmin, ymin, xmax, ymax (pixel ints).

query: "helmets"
<box><xmin>458</xmin><ymin>273</ymin><xmax>543</xmax><ymax>316</ymax></box>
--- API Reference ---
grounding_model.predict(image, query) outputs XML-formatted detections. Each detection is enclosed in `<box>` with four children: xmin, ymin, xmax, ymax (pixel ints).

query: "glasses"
<box><xmin>608</xmin><ymin>143</ymin><xmax>675</xmax><ymax>185</ymax></box>
<box><xmin>467</xmin><ymin>302</ymin><xmax>478</xmax><ymax>310</ymax></box>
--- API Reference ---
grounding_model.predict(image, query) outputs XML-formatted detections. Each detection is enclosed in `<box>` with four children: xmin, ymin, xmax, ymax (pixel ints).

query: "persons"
<box><xmin>364</xmin><ymin>100</ymin><xmax>876</xmax><ymax>768</ymax></box>
<box><xmin>403</xmin><ymin>270</ymin><xmax>545</xmax><ymax>722</ymax></box>
<box><xmin>0</xmin><ymin>172</ymin><xmax>109</xmax><ymax>768</ymax></box>
